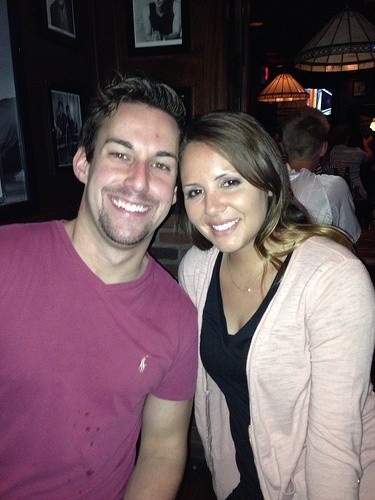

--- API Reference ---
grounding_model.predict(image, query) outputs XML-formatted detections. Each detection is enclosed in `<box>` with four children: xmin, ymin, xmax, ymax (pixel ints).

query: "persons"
<box><xmin>262</xmin><ymin>112</ymin><xmax>375</xmax><ymax>230</ymax></box>
<box><xmin>52</xmin><ymin>97</ymin><xmax>80</xmax><ymax>149</ymax></box>
<box><xmin>0</xmin><ymin>75</ymin><xmax>199</xmax><ymax>500</ymax></box>
<box><xmin>178</xmin><ymin>110</ymin><xmax>375</xmax><ymax>499</ymax></box>
<box><xmin>276</xmin><ymin>107</ymin><xmax>364</xmax><ymax>246</ymax></box>
<box><xmin>142</xmin><ymin>0</ymin><xmax>181</xmax><ymax>40</ymax></box>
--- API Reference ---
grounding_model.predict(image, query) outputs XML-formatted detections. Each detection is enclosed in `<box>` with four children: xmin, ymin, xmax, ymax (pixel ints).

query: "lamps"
<box><xmin>256</xmin><ymin>69</ymin><xmax>311</xmax><ymax>105</ymax></box>
<box><xmin>295</xmin><ymin>10</ymin><xmax>375</xmax><ymax>73</ymax></box>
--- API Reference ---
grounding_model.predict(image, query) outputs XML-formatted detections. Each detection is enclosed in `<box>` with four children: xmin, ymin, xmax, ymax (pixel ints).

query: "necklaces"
<box><xmin>227</xmin><ymin>251</ymin><xmax>273</xmax><ymax>293</ymax></box>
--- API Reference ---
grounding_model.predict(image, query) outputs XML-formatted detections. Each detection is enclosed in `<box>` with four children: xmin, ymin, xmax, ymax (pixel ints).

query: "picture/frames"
<box><xmin>41</xmin><ymin>79</ymin><xmax>87</xmax><ymax>176</ymax></box>
<box><xmin>0</xmin><ymin>0</ymin><xmax>41</xmax><ymax>220</ymax></box>
<box><xmin>41</xmin><ymin>0</ymin><xmax>78</xmax><ymax>44</ymax></box>
<box><xmin>125</xmin><ymin>0</ymin><xmax>194</xmax><ymax>55</ymax></box>
<box><xmin>352</xmin><ymin>79</ymin><xmax>368</xmax><ymax>97</ymax></box>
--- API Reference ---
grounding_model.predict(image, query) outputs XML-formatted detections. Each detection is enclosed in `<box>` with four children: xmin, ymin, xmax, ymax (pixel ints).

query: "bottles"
<box><xmin>333</xmin><ymin>168</ymin><xmax>338</xmax><ymax>175</ymax></box>
<box><xmin>342</xmin><ymin>167</ymin><xmax>351</xmax><ymax>192</ymax></box>
